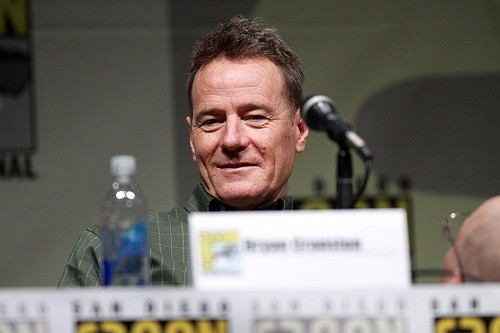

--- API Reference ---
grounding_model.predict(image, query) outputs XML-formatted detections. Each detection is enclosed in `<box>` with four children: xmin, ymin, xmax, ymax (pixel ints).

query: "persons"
<box><xmin>440</xmin><ymin>194</ymin><xmax>500</xmax><ymax>284</ymax></box>
<box><xmin>59</xmin><ymin>16</ymin><xmax>310</xmax><ymax>288</ymax></box>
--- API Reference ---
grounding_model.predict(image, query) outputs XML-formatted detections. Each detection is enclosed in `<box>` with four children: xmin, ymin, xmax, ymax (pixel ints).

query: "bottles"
<box><xmin>101</xmin><ymin>156</ymin><xmax>152</xmax><ymax>286</ymax></box>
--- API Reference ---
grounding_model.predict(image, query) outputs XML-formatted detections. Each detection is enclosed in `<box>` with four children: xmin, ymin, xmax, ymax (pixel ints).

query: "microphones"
<box><xmin>300</xmin><ymin>96</ymin><xmax>372</xmax><ymax>161</ymax></box>
